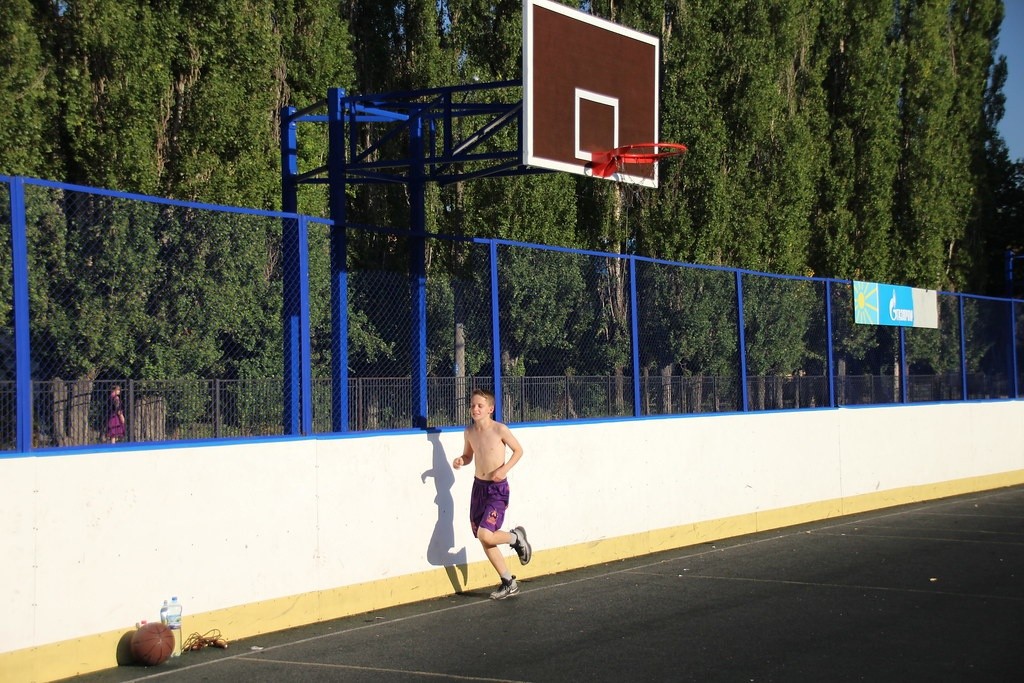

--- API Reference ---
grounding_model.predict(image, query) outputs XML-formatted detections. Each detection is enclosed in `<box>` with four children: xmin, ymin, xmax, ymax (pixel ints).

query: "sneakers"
<box><xmin>510</xmin><ymin>526</ymin><xmax>531</xmax><ymax>565</ymax></box>
<box><xmin>490</xmin><ymin>575</ymin><xmax>519</xmax><ymax>600</ymax></box>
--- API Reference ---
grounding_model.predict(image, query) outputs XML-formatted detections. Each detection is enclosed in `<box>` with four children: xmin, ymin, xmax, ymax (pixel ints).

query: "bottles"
<box><xmin>141</xmin><ymin>619</ymin><xmax>147</xmax><ymax>627</ymax></box>
<box><xmin>167</xmin><ymin>597</ymin><xmax>182</xmax><ymax>657</ymax></box>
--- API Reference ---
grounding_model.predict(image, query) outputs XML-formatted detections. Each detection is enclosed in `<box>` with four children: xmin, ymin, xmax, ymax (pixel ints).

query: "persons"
<box><xmin>104</xmin><ymin>384</ymin><xmax>125</xmax><ymax>444</ymax></box>
<box><xmin>453</xmin><ymin>390</ymin><xmax>533</xmax><ymax>600</ymax></box>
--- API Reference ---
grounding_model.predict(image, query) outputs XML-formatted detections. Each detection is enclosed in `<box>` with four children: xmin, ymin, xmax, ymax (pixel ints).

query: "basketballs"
<box><xmin>130</xmin><ymin>622</ymin><xmax>176</xmax><ymax>667</ymax></box>
